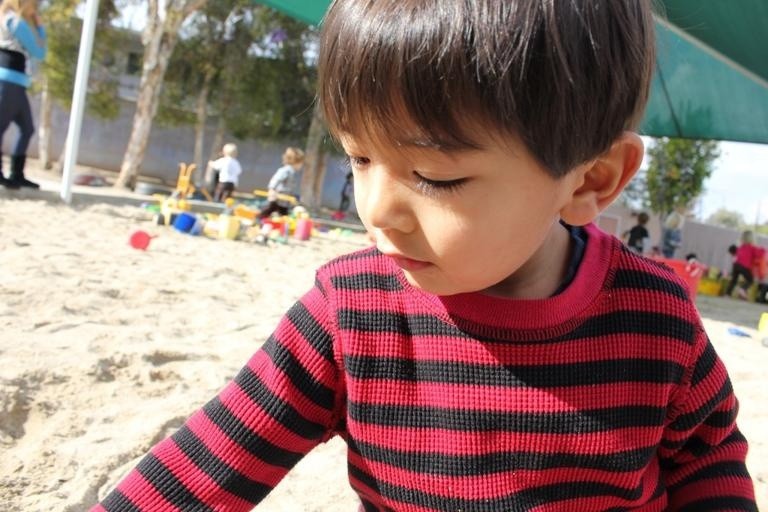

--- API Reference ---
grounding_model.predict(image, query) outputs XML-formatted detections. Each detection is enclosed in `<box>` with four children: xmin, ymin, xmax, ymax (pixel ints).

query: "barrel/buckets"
<box><xmin>655</xmin><ymin>258</ymin><xmax>706</xmax><ymax>303</ymax></box>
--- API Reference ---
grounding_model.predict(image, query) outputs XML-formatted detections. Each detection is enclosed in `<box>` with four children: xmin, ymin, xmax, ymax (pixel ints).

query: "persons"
<box><xmin>208</xmin><ymin>143</ymin><xmax>242</xmax><ymax>203</ymax></box>
<box><xmin>254</xmin><ymin>146</ymin><xmax>306</xmax><ymax>225</ymax></box>
<box><xmin>620</xmin><ymin>211</ymin><xmax>768</xmax><ymax>306</ymax></box>
<box><xmin>0</xmin><ymin>0</ymin><xmax>49</xmax><ymax>190</ymax></box>
<box><xmin>339</xmin><ymin>173</ymin><xmax>354</xmax><ymax>213</ymax></box>
<box><xmin>80</xmin><ymin>0</ymin><xmax>758</xmax><ymax>512</ymax></box>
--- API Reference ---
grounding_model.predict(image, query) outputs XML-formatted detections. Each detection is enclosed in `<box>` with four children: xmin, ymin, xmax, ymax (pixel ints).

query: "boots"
<box><xmin>0</xmin><ymin>152</ymin><xmax>40</xmax><ymax>189</ymax></box>
